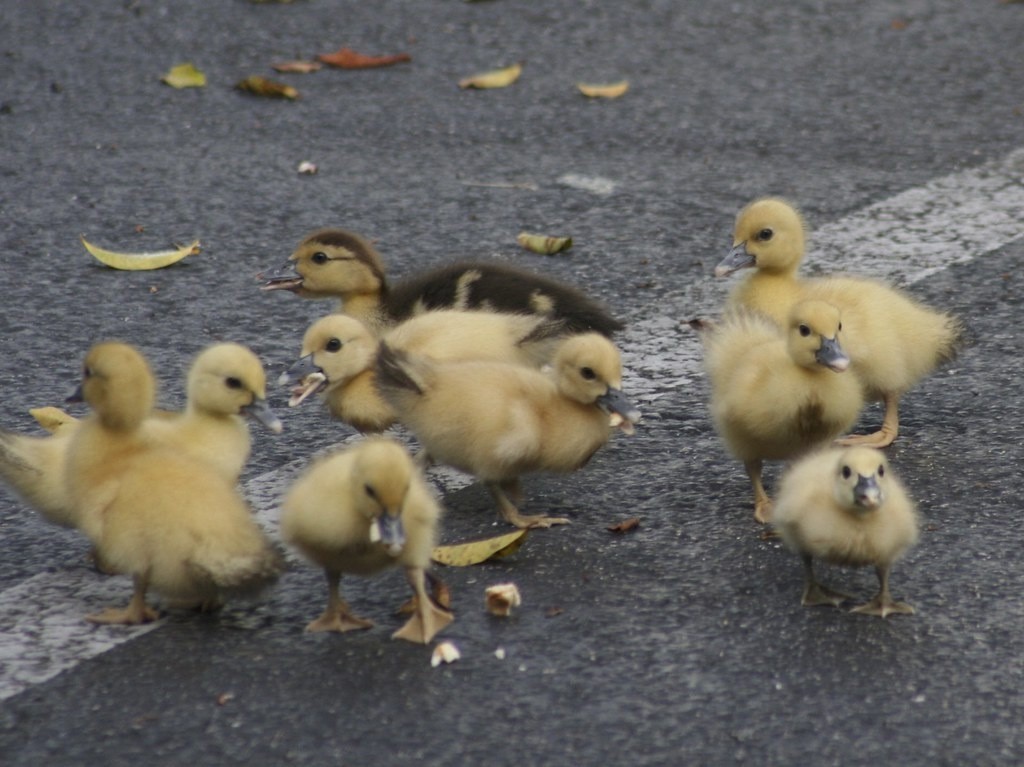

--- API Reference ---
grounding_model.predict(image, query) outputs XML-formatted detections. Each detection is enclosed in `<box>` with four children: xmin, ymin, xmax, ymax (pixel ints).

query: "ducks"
<box><xmin>0</xmin><ymin>228</ymin><xmax>643</xmax><ymax>645</ymax></box>
<box><xmin>705</xmin><ymin>195</ymin><xmax>963</xmax><ymax>616</ymax></box>
